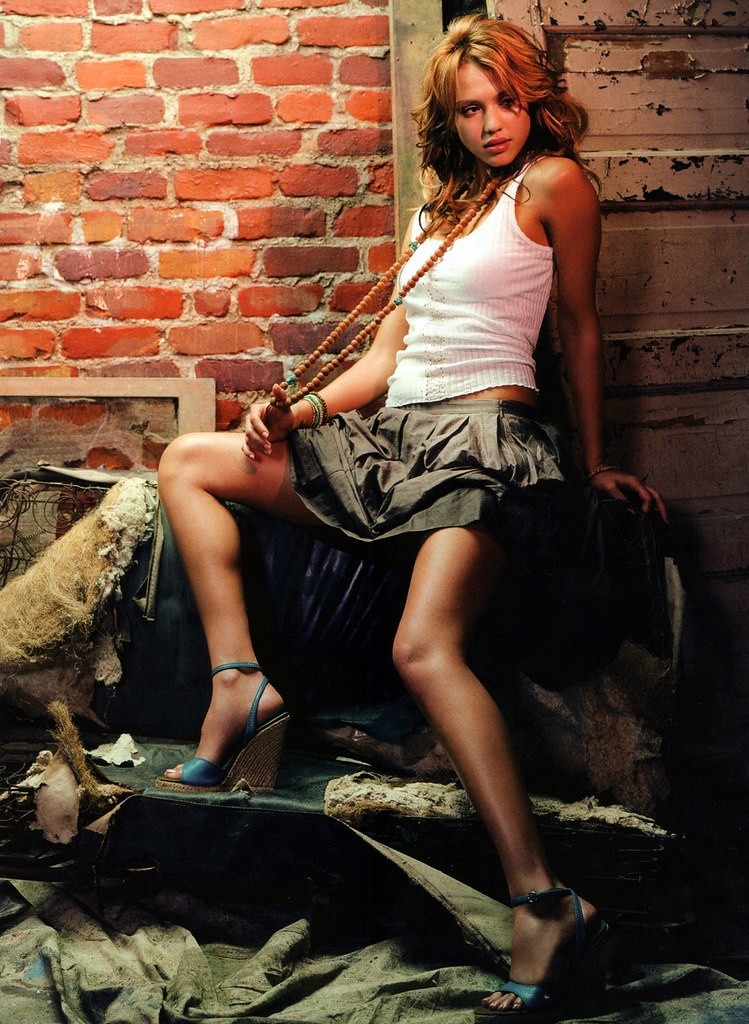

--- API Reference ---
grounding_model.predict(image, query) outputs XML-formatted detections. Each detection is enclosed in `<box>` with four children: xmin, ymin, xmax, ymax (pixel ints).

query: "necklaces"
<box><xmin>269</xmin><ymin>171</ymin><xmax>503</xmax><ymax>406</ymax></box>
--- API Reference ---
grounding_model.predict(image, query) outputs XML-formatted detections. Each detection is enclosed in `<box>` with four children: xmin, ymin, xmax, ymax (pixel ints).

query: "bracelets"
<box><xmin>302</xmin><ymin>391</ymin><xmax>328</xmax><ymax>429</ymax></box>
<box><xmin>589</xmin><ymin>465</ymin><xmax>619</xmax><ymax>478</ymax></box>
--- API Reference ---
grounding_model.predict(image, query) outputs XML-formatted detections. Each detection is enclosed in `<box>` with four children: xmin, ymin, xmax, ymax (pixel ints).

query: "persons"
<box><xmin>157</xmin><ymin>12</ymin><xmax>669</xmax><ymax>1024</ymax></box>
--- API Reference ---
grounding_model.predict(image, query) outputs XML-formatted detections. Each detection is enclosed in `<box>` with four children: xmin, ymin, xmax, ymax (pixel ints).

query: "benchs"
<box><xmin>0</xmin><ymin>477</ymin><xmax>727</xmax><ymax>962</ymax></box>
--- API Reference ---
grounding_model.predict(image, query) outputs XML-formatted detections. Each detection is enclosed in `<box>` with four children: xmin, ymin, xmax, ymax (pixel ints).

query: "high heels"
<box><xmin>471</xmin><ymin>882</ymin><xmax>614</xmax><ymax>1024</ymax></box>
<box><xmin>156</xmin><ymin>661</ymin><xmax>290</xmax><ymax>795</ymax></box>
